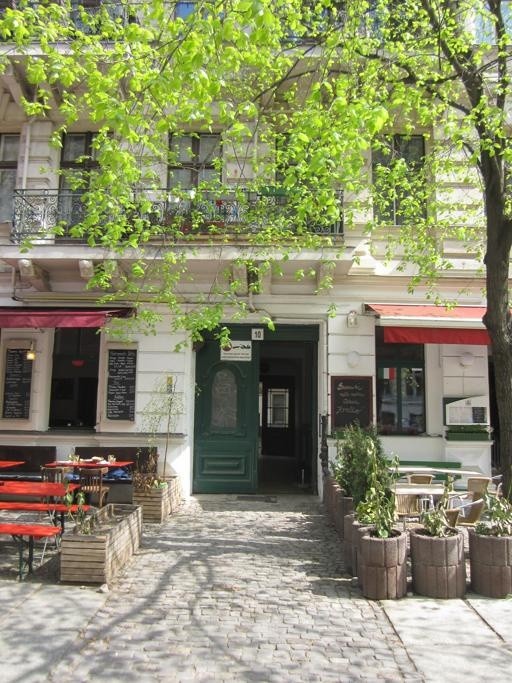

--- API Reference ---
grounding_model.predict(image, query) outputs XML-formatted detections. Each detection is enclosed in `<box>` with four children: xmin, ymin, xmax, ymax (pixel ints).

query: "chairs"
<box><xmin>409</xmin><ymin>473</ymin><xmax>489</xmax><ymax>526</ymax></box>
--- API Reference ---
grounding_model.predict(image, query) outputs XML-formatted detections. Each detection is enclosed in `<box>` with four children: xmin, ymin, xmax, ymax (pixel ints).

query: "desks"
<box><xmin>390</xmin><ymin>484</ymin><xmax>458</xmax><ymax>517</ymax></box>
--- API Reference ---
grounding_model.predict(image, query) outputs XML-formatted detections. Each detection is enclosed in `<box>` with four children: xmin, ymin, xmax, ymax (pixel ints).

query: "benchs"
<box><xmin>0</xmin><ymin>498</ymin><xmax>92</xmax><ymax>580</ymax></box>
<box><xmin>0</xmin><ymin>445</ymin><xmax>154</xmax><ymax>503</ymax></box>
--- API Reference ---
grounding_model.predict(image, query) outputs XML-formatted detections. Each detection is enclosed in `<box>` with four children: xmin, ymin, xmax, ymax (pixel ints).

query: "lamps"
<box><xmin>25</xmin><ymin>337</ymin><xmax>43</xmax><ymax>360</ymax></box>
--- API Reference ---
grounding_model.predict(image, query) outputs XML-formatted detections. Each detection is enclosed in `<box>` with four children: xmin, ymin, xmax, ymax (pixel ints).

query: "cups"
<box><xmin>72</xmin><ymin>455</ymin><xmax>79</xmax><ymax>464</ymax></box>
<box><xmin>108</xmin><ymin>455</ymin><xmax>114</xmax><ymax>465</ymax></box>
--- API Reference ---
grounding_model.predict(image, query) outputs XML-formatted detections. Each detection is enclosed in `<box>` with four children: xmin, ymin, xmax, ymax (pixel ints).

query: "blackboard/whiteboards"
<box><xmin>103</xmin><ymin>341</ymin><xmax>139</xmax><ymax>424</ymax></box>
<box><xmin>331</xmin><ymin>376</ymin><xmax>373</xmax><ymax>439</ymax></box>
<box><xmin>0</xmin><ymin>338</ymin><xmax>37</xmax><ymax>423</ymax></box>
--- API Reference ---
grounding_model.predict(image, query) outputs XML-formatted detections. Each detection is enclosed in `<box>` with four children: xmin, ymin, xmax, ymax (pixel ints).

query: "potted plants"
<box><xmin>329</xmin><ymin>419</ymin><xmax>403</xmax><ymax>602</ymax></box>
<box><xmin>61</xmin><ymin>477</ymin><xmax>143</xmax><ymax>584</ymax></box>
<box><xmin>411</xmin><ymin>498</ymin><xmax>511</xmax><ymax>599</ymax></box>
<box><xmin>136</xmin><ymin>369</ymin><xmax>188</xmax><ymax>518</ymax></box>
